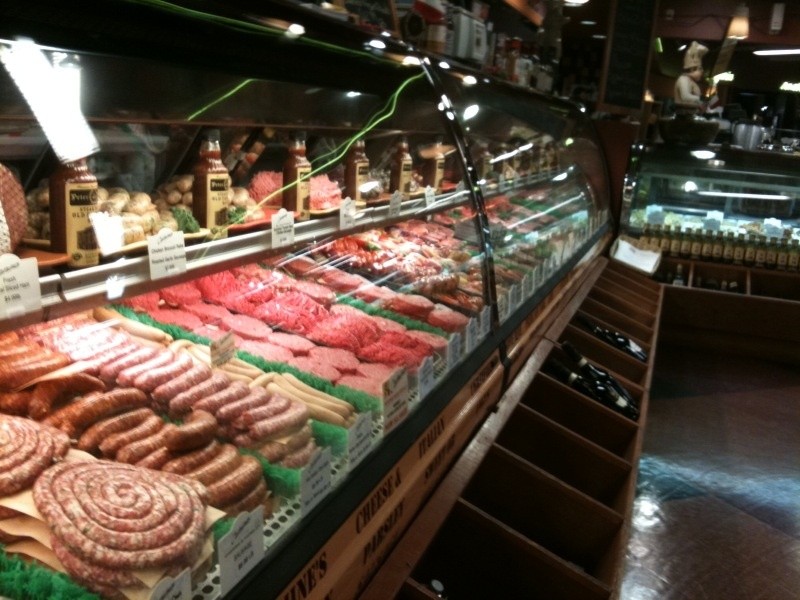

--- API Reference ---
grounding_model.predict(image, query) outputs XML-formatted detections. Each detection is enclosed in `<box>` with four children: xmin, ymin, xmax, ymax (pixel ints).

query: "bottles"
<box><xmin>49</xmin><ymin>158</ymin><xmax>99</xmax><ymax>272</ymax></box>
<box><xmin>510</xmin><ymin>141</ymin><xmax>556</xmax><ymax>172</ymax></box>
<box><xmin>640</xmin><ymin>224</ymin><xmax>692</xmax><ymax>259</ymax></box>
<box><xmin>495</xmin><ymin>142</ymin><xmax>508</xmax><ymax>173</ymax></box>
<box><xmin>765</xmin><ymin>237</ymin><xmax>777</xmax><ymax>269</ymax></box>
<box><xmin>389</xmin><ymin>134</ymin><xmax>412</xmax><ymax>195</ymax></box>
<box><xmin>722</xmin><ymin>232</ymin><xmax>734</xmax><ymax>263</ymax></box>
<box><xmin>486</xmin><ymin>22</ymin><xmax>556</xmax><ymax>94</ymax></box>
<box><xmin>667</xmin><ymin>263</ymin><xmax>684</xmax><ymax>286</ymax></box>
<box><xmin>787</xmin><ymin>239</ymin><xmax>800</xmax><ymax>272</ymax></box>
<box><xmin>194</xmin><ymin>128</ymin><xmax>229</xmax><ymax>228</ymax></box>
<box><xmin>552</xmin><ymin>341</ymin><xmax>640</xmax><ymax>422</ymax></box>
<box><xmin>478</xmin><ymin>142</ymin><xmax>494</xmax><ymax>180</ymax></box>
<box><xmin>423</xmin><ymin>134</ymin><xmax>445</xmax><ymax>190</ymax></box>
<box><xmin>701</xmin><ymin>230</ymin><xmax>712</xmax><ymax>262</ymax></box>
<box><xmin>777</xmin><ymin>238</ymin><xmax>788</xmax><ymax>271</ymax></box>
<box><xmin>712</xmin><ymin>231</ymin><xmax>722</xmax><ymax>263</ymax></box>
<box><xmin>575</xmin><ymin>313</ymin><xmax>647</xmax><ymax>362</ymax></box>
<box><xmin>733</xmin><ymin>234</ymin><xmax>745</xmax><ymax>264</ymax></box>
<box><xmin>426</xmin><ymin>17</ymin><xmax>455</xmax><ymax>55</ymax></box>
<box><xmin>690</xmin><ymin>229</ymin><xmax>701</xmax><ymax>260</ymax></box>
<box><xmin>743</xmin><ymin>235</ymin><xmax>756</xmax><ymax>265</ymax></box>
<box><xmin>346</xmin><ymin>133</ymin><xmax>368</xmax><ymax>201</ymax></box>
<box><xmin>283</xmin><ymin>130</ymin><xmax>311</xmax><ymax>222</ymax></box>
<box><xmin>755</xmin><ymin>237</ymin><xmax>766</xmax><ymax>267</ymax></box>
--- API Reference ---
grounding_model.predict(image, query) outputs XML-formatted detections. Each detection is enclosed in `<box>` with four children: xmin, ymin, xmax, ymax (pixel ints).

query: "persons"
<box><xmin>672</xmin><ymin>43</ymin><xmax>711</xmax><ymax>106</ymax></box>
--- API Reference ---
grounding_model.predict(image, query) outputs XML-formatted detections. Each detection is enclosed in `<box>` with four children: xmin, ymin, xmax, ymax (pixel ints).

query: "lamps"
<box><xmin>726</xmin><ymin>0</ymin><xmax>751</xmax><ymax>40</ymax></box>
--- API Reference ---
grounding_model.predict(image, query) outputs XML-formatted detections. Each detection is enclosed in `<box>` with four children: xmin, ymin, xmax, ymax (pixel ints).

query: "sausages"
<box><xmin>0</xmin><ymin>306</ymin><xmax>360</xmax><ymax>600</ymax></box>
<box><xmin>21</xmin><ymin>173</ymin><xmax>267</xmax><ymax>248</ymax></box>
